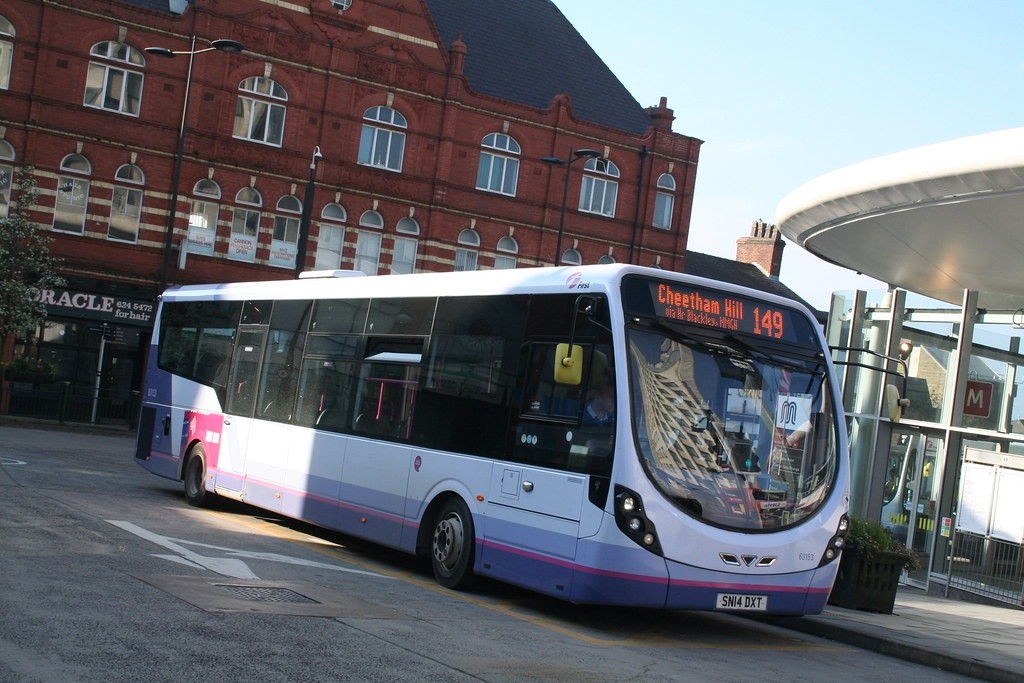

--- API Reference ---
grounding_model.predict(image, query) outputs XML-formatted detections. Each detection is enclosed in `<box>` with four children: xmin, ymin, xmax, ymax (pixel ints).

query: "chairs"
<box><xmin>178</xmin><ymin>351</ymin><xmax>407</xmax><ymax>439</ymax></box>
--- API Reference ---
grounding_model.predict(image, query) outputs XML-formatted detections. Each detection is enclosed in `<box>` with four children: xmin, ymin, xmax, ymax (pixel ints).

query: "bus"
<box><xmin>135</xmin><ymin>264</ymin><xmax>911</xmax><ymax>617</ymax></box>
<box><xmin>879</xmin><ymin>444</ymin><xmax>936</xmax><ymax>548</ymax></box>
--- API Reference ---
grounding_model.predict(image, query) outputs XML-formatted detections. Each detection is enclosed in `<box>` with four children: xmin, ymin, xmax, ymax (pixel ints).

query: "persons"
<box><xmin>885</xmin><ymin>468</ymin><xmax>909</xmax><ymax>503</ymax></box>
<box><xmin>572</xmin><ymin>383</ymin><xmax>616</xmax><ymax>455</ymax></box>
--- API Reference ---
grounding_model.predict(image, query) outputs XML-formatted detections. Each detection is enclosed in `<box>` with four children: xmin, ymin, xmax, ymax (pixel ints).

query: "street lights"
<box><xmin>294</xmin><ymin>143</ymin><xmax>324</xmax><ymax>281</ymax></box>
<box><xmin>540</xmin><ymin>148</ymin><xmax>603</xmax><ymax>267</ymax></box>
<box><xmin>143</xmin><ymin>34</ymin><xmax>247</xmax><ymax>295</ymax></box>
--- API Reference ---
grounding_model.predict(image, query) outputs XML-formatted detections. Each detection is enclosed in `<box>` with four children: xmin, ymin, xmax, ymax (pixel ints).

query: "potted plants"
<box><xmin>827</xmin><ymin>517</ymin><xmax>921</xmax><ymax>612</ymax></box>
